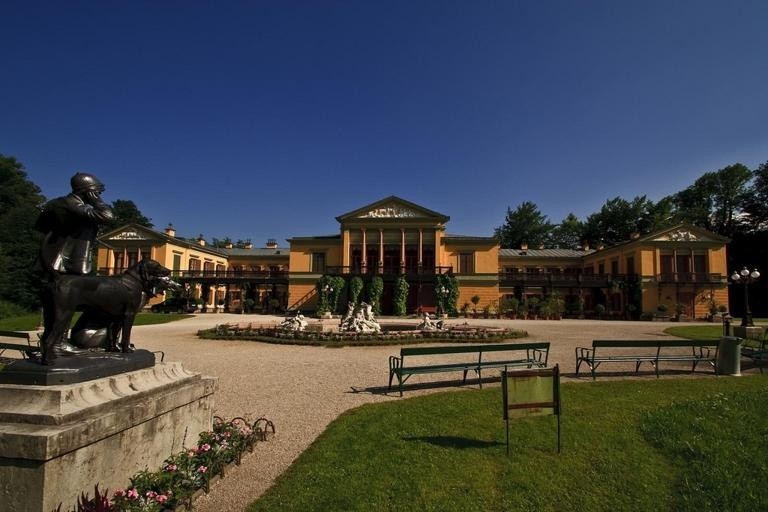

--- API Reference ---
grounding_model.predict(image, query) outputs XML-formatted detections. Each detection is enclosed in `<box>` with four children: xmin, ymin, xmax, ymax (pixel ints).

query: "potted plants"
<box><xmin>460</xmin><ymin>289</ymin><xmax>566</xmax><ymax>320</ymax></box>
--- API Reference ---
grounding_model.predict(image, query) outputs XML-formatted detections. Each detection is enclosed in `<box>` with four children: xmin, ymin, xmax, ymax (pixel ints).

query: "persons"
<box><xmin>417</xmin><ymin>311</ymin><xmax>437</xmax><ymax>330</ymax></box>
<box><xmin>280</xmin><ymin>310</ymin><xmax>308</xmax><ymax>331</ymax></box>
<box><xmin>341</xmin><ymin>301</ymin><xmax>381</xmax><ymax>333</ymax></box>
<box><xmin>35</xmin><ymin>172</ymin><xmax>114</xmax><ymax>357</ymax></box>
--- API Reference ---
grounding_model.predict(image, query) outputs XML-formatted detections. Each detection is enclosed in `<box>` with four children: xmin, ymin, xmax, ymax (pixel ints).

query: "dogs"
<box><xmin>38</xmin><ymin>258</ymin><xmax>183</xmax><ymax>367</ymax></box>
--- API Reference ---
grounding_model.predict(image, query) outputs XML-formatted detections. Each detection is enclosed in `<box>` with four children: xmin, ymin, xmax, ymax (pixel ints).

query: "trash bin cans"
<box><xmin>718</xmin><ymin>336</ymin><xmax>743</xmax><ymax>374</ymax></box>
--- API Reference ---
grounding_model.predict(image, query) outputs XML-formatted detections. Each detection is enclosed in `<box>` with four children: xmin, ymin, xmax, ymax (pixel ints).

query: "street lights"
<box><xmin>185</xmin><ymin>285</ymin><xmax>190</xmax><ymax>309</ymax></box>
<box><xmin>321</xmin><ymin>285</ymin><xmax>333</xmax><ymax>312</ymax></box>
<box><xmin>441</xmin><ymin>287</ymin><xmax>449</xmax><ymax>314</ymax></box>
<box><xmin>731</xmin><ymin>267</ymin><xmax>760</xmax><ymax>327</ymax></box>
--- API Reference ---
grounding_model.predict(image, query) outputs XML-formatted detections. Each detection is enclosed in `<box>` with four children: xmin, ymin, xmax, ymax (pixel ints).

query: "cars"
<box><xmin>150</xmin><ymin>298</ymin><xmax>198</xmax><ymax>314</ymax></box>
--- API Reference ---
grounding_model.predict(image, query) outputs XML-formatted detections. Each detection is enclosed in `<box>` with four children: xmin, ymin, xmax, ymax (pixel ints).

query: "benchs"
<box><xmin>740</xmin><ymin>329</ymin><xmax>766</xmax><ymax>374</ymax></box>
<box><xmin>574</xmin><ymin>336</ymin><xmax>721</xmax><ymax>379</ymax></box>
<box><xmin>386</xmin><ymin>341</ymin><xmax>550</xmax><ymax>396</ymax></box>
